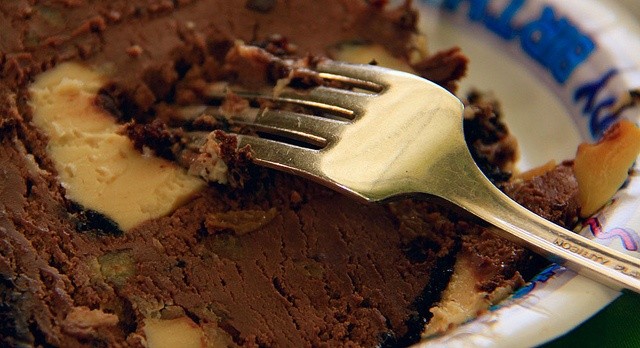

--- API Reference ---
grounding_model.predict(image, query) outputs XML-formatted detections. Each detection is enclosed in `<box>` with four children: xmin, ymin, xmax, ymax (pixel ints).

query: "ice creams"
<box><xmin>1</xmin><ymin>1</ymin><xmax>640</xmax><ymax>348</ymax></box>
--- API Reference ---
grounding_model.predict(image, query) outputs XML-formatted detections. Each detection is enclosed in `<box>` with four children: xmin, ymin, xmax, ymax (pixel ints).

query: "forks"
<box><xmin>173</xmin><ymin>61</ymin><xmax>640</xmax><ymax>296</ymax></box>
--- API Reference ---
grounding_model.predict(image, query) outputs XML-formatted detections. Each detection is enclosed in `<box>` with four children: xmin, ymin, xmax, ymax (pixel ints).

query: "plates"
<box><xmin>411</xmin><ymin>1</ymin><xmax>640</xmax><ymax>347</ymax></box>
